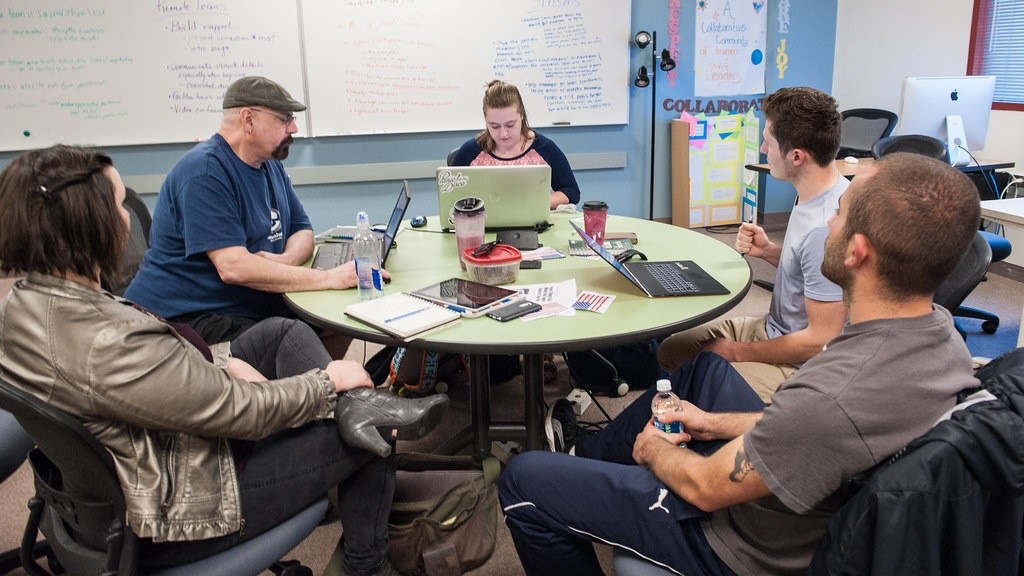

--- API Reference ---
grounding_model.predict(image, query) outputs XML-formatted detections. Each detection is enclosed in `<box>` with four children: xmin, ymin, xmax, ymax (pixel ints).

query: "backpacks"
<box><xmin>386</xmin><ymin>342</ymin><xmax>473</xmax><ymax>396</ymax></box>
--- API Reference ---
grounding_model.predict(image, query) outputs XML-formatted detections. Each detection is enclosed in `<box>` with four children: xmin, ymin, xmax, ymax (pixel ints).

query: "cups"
<box><xmin>451</xmin><ymin>198</ymin><xmax>485</xmax><ymax>271</ymax></box>
<box><xmin>582</xmin><ymin>201</ymin><xmax>609</xmax><ymax>248</ymax></box>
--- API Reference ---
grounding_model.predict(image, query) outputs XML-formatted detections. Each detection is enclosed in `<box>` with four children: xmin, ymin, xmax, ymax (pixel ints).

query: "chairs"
<box><xmin>835</xmin><ymin>108</ymin><xmax>899</xmax><ymax>160</ymax></box>
<box><xmin>100</xmin><ymin>187</ymin><xmax>152</xmax><ymax>293</ymax></box>
<box><xmin>871</xmin><ymin>134</ymin><xmax>1012</xmax><ymax>342</ymax></box>
<box><xmin>613</xmin><ymin>344</ymin><xmax>1024</xmax><ymax>576</ymax></box>
<box><xmin>0</xmin><ymin>366</ymin><xmax>329</xmax><ymax>576</ymax></box>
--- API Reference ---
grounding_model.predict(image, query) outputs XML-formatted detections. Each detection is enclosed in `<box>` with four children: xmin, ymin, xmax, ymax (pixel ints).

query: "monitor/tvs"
<box><xmin>896</xmin><ymin>76</ymin><xmax>996</xmax><ymax>166</ymax></box>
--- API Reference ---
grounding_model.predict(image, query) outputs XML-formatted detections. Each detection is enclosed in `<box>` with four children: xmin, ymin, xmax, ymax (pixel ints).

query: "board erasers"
<box><xmin>604</xmin><ymin>232</ymin><xmax>638</xmax><ymax>245</ymax></box>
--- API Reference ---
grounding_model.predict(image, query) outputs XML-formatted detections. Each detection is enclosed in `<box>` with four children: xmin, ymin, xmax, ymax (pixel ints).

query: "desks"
<box><xmin>743</xmin><ymin>155</ymin><xmax>1016</xmax><ymax>293</ymax></box>
<box><xmin>978</xmin><ymin>197</ymin><xmax>1024</xmax><ymax>350</ymax></box>
<box><xmin>281</xmin><ymin>209</ymin><xmax>755</xmax><ymax>459</ymax></box>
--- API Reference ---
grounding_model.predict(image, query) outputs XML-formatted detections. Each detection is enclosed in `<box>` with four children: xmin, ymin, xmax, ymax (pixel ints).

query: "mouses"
<box><xmin>436</xmin><ymin>165</ymin><xmax>552</xmax><ymax>234</ymax></box>
<box><xmin>382</xmin><ymin>276</ymin><xmax>391</xmax><ymax>284</ymax></box>
<box><xmin>410</xmin><ymin>215</ymin><xmax>427</xmax><ymax>229</ymax></box>
<box><xmin>844</xmin><ymin>156</ymin><xmax>858</xmax><ymax>164</ymax></box>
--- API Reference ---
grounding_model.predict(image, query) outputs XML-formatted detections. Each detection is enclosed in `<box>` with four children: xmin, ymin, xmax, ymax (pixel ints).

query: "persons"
<box><xmin>0</xmin><ymin>144</ymin><xmax>449</xmax><ymax>576</ymax></box>
<box><xmin>498</xmin><ymin>152</ymin><xmax>982</xmax><ymax>576</ymax></box>
<box><xmin>451</xmin><ymin>80</ymin><xmax>580</xmax><ymax>378</ymax></box>
<box><xmin>122</xmin><ymin>77</ymin><xmax>391</xmax><ymax>361</ymax></box>
<box><xmin>656</xmin><ymin>88</ymin><xmax>851</xmax><ymax>404</ymax></box>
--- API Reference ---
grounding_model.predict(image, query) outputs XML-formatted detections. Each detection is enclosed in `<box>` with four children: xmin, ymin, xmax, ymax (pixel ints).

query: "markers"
<box><xmin>552</xmin><ymin>119</ymin><xmax>574</xmax><ymax>127</ymax></box>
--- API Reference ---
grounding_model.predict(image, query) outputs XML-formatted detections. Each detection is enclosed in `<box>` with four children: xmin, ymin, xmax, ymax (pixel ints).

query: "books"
<box><xmin>344</xmin><ymin>290</ymin><xmax>463</xmax><ymax>342</ymax></box>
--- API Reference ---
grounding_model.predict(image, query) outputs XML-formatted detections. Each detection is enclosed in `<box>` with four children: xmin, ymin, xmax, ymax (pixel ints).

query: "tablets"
<box><xmin>410</xmin><ymin>278</ymin><xmax>518</xmax><ymax>313</ymax></box>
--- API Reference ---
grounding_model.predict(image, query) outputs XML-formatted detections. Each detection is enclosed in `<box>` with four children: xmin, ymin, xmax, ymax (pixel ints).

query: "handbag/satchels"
<box><xmin>566</xmin><ymin>333</ymin><xmax>671</xmax><ymax>395</ymax></box>
<box><xmin>385</xmin><ymin>475</ymin><xmax>499</xmax><ymax>576</ymax></box>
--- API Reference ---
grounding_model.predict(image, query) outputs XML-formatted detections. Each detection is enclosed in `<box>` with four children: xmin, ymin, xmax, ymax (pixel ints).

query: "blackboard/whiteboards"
<box><xmin>0</xmin><ymin>0</ymin><xmax>631</xmax><ymax>152</ymax></box>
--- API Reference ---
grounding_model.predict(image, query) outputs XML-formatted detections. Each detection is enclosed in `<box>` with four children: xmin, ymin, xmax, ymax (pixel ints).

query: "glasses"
<box><xmin>239</xmin><ymin>109</ymin><xmax>296</xmax><ymax>125</ymax></box>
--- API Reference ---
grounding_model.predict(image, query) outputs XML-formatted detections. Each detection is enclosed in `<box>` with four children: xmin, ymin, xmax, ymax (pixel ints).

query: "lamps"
<box><xmin>634</xmin><ymin>30</ymin><xmax>676</xmax><ymax>220</ymax></box>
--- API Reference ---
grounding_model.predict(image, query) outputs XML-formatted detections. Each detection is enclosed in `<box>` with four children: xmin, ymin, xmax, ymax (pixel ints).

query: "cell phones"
<box><xmin>486</xmin><ymin>301</ymin><xmax>543</xmax><ymax>322</ymax></box>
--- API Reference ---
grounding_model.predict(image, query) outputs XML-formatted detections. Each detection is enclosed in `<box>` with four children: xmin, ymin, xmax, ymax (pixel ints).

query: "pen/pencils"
<box><xmin>384</xmin><ymin>306</ymin><xmax>430</xmax><ymax>323</ymax></box>
<box><xmin>327</xmin><ymin>234</ymin><xmax>353</xmax><ymax>240</ymax></box>
<box><xmin>331</xmin><ymin>234</ymin><xmax>351</xmax><ymax>241</ymax></box>
<box><xmin>371</xmin><ymin>227</ymin><xmax>387</xmax><ymax>233</ymax></box>
<box><xmin>741</xmin><ymin>217</ymin><xmax>753</xmax><ymax>258</ymax></box>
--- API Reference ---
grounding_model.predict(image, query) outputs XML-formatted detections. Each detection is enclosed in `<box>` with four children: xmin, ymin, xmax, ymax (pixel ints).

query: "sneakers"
<box><xmin>545</xmin><ymin>397</ymin><xmax>581</xmax><ymax>455</ymax></box>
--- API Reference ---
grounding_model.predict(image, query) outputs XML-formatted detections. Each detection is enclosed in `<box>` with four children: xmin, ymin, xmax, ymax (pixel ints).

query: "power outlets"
<box><xmin>1009</xmin><ymin>175</ymin><xmax>1024</xmax><ymax>187</ymax></box>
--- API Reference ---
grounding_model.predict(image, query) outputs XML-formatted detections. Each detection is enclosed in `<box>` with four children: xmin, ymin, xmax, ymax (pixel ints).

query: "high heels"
<box><xmin>334</xmin><ymin>386</ymin><xmax>450</xmax><ymax>457</ymax></box>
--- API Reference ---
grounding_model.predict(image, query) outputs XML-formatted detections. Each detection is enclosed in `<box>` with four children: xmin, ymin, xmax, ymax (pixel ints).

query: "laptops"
<box><xmin>569</xmin><ymin>220</ymin><xmax>730</xmax><ymax>298</ymax></box>
<box><xmin>311</xmin><ymin>180</ymin><xmax>411</xmax><ymax>272</ymax></box>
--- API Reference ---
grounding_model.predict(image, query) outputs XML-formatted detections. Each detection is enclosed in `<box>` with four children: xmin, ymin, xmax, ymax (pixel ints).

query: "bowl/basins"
<box><xmin>461</xmin><ymin>245</ymin><xmax>522</xmax><ymax>285</ymax></box>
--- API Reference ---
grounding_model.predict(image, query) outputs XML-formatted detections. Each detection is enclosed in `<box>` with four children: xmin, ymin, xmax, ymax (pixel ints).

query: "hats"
<box><xmin>222</xmin><ymin>76</ymin><xmax>307</xmax><ymax>112</ymax></box>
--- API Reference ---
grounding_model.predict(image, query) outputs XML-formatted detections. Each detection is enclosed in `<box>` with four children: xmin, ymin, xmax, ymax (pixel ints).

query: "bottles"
<box><xmin>353</xmin><ymin>212</ymin><xmax>384</xmax><ymax>301</ymax></box>
<box><xmin>651</xmin><ymin>379</ymin><xmax>682</xmax><ymax>447</ymax></box>
